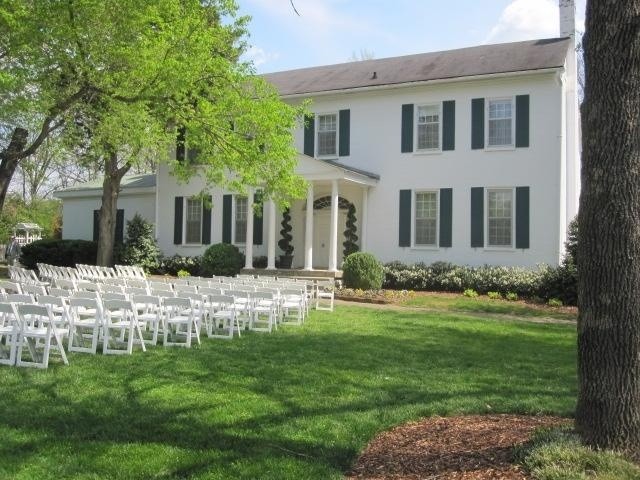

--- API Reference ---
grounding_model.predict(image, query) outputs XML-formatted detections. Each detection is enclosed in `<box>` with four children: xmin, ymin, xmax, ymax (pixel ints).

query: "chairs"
<box><xmin>1</xmin><ymin>263</ymin><xmax>336</xmax><ymax>369</ymax></box>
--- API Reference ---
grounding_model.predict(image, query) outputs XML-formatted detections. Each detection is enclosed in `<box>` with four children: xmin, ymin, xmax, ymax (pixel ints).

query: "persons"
<box><xmin>5</xmin><ymin>236</ymin><xmax>21</xmax><ymax>278</ymax></box>
